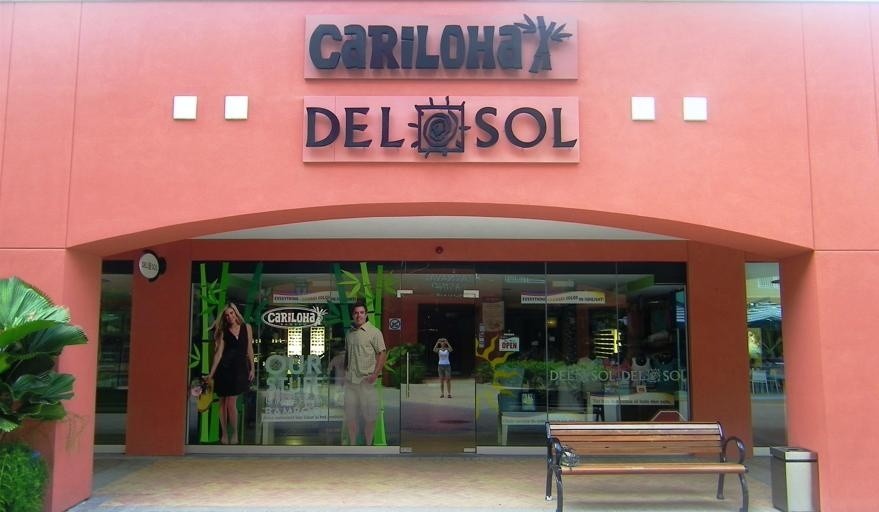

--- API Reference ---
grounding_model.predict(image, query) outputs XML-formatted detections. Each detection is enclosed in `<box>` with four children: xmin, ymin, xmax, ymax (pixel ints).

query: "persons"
<box><xmin>203</xmin><ymin>302</ymin><xmax>255</xmax><ymax>445</ymax></box>
<box><xmin>477</xmin><ymin>336</ymin><xmax>685</xmax><ymax>423</ymax></box>
<box><xmin>749</xmin><ymin>357</ymin><xmax>756</xmax><ymax>369</ymax></box>
<box><xmin>433</xmin><ymin>338</ymin><xmax>454</xmax><ymax>398</ymax></box>
<box><xmin>344</xmin><ymin>303</ymin><xmax>387</xmax><ymax>446</ymax></box>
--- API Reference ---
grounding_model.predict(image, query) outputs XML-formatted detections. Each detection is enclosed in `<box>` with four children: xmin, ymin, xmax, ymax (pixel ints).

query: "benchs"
<box><xmin>497</xmin><ymin>412</ymin><xmax>595</xmax><ymax>446</ymax></box>
<box><xmin>262</xmin><ymin>408</ymin><xmax>344</xmax><ymax>445</ymax></box>
<box><xmin>545</xmin><ymin>421</ymin><xmax>749</xmax><ymax>512</ymax></box>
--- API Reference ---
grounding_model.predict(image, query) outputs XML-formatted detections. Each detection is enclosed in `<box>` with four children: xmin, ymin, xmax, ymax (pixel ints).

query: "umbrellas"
<box><xmin>747</xmin><ymin>301</ymin><xmax>783</xmax><ymax>359</ymax></box>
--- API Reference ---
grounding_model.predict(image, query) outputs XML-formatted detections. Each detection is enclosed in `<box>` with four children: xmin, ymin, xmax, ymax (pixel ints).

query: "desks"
<box><xmin>586</xmin><ymin>391</ymin><xmax>689</xmax><ymax>422</ymax></box>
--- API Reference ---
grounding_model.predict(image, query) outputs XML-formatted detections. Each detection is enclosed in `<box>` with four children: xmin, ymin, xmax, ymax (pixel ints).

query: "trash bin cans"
<box><xmin>770</xmin><ymin>446</ymin><xmax>820</xmax><ymax>512</ymax></box>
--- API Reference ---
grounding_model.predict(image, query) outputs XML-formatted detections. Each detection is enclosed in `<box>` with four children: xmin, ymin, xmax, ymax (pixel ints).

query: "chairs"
<box><xmin>752</xmin><ymin>368</ymin><xmax>780</xmax><ymax>395</ymax></box>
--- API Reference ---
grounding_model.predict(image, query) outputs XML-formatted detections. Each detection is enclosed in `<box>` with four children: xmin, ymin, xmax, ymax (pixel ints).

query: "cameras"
<box><xmin>440</xmin><ymin>339</ymin><xmax>445</xmax><ymax>342</ymax></box>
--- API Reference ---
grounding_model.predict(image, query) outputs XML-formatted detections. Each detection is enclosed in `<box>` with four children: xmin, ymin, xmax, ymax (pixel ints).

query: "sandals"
<box><xmin>440</xmin><ymin>394</ymin><xmax>444</xmax><ymax>398</ymax></box>
<box><xmin>448</xmin><ymin>394</ymin><xmax>451</xmax><ymax>398</ymax></box>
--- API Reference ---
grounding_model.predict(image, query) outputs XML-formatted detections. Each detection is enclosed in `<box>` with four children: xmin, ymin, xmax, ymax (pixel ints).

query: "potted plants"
<box><xmin>493</xmin><ymin>359</ymin><xmax>565</xmax><ymax>412</ymax></box>
